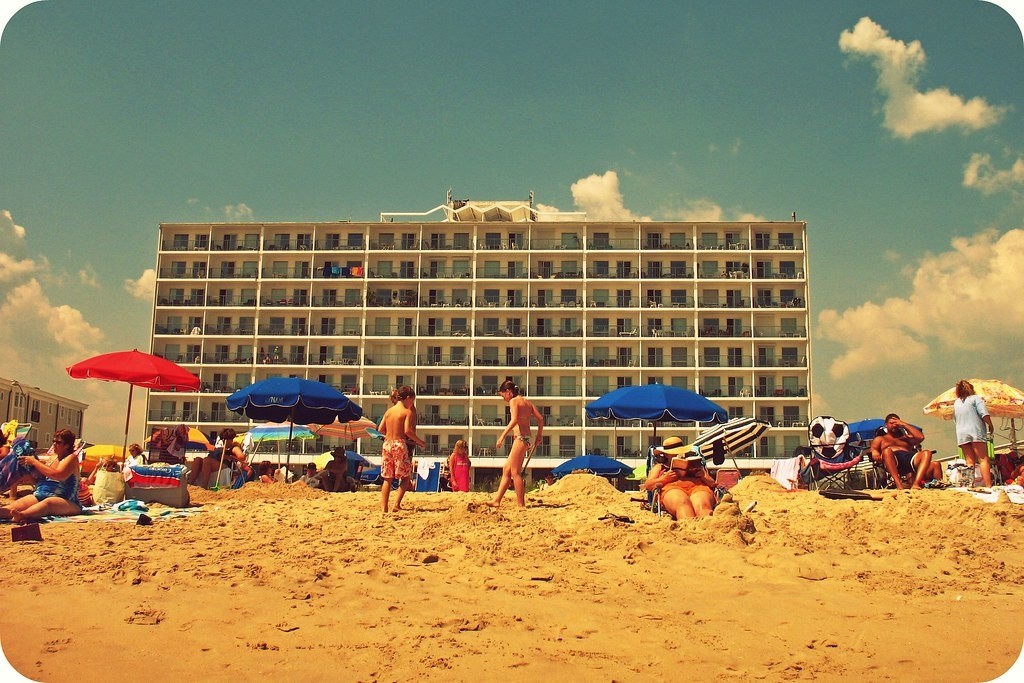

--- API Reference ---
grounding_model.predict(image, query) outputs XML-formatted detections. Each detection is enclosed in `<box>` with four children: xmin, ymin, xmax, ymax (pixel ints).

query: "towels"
<box><xmin>417</xmin><ymin>460</ymin><xmax>436</xmax><ymax>480</ymax></box>
<box><xmin>112</xmin><ymin>498</ymin><xmax>150</xmax><ymax>514</ymax></box>
<box><xmin>123</xmin><ymin>462</ymin><xmax>187</xmax><ymax>490</ymax></box>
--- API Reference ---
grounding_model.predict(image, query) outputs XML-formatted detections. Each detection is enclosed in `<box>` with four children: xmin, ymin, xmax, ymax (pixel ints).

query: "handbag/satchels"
<box><xmin>91</xmin><ymin>461</ymin><xmax>125</xmax><ymax>506</ymax></box>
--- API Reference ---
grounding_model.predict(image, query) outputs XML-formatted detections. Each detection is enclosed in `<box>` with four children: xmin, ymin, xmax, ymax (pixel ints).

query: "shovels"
<box><xmin>209</xmin><ymin>440</ymin><xmax>226</xmax><ymax>492</ymax></box>
<box><xmin>520</xmin><ymin>443</ymin><xmax>537</xmax><ymax>479</ymax></box>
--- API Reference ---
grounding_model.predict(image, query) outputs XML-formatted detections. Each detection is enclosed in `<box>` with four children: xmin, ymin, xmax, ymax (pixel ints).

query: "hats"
<box><xmin>331</xmin><ymin>447</ymin><xmax>347</xmax><ymax>460</ymax></box>
<box><xmin>654</xmin><ymin>436</ymin><xmax>693</xmax><ymax>456</ymax></box>
<box><xmin>307</xmin><ymin>462</ymin><xmax>317</xmax><ymax>469</ymax></box>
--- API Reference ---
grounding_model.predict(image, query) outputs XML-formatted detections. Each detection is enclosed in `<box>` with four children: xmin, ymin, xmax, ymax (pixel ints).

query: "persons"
<box><xmin>450</xmin><ymin>439</ymin><xmax>471</xmax><ymax>493</ymax></box>
<box><xmin>295</xmin><ymin>443</ymin><xmax>356</xmax><ymax>495</ymax></box>
<box><xmin>0</xmin><ymin>424</ymin><xmax>189</xmax><ymax>524</ymax></box>
<box><xmin>189</xmin><ymin>427</ymin><xmax>276</xmax><ymax>489</ymax></box>
<box><xmin>954</xmin><ymin>379</ymin><xmax>994</xmax><ymax>488</ymax></box>
<box><xmin>645</xmin><ymin>437</ymin><xmax>719</xmax><ymax>520</ymax></box>
<box><xmin>274</xmin><ymin>345</ymin><xmax>280</xmax><ymax>365</ymax></box>
<box><xmin>377</xmin><ymin>385</ymin><xmax>427</xmax><ymax>514</ymax></box>
<box><xmin>538</xmin><ymin>472</ymin><xmax>556</xmax><ymax>492</ymax></box>
<box><xmin>485</xmin><ymin>380</ymin><xmax>543</xmax><ymax>506</ymax></box>
<box><xmin>265</xmin><ymin>354</ymin><xmax>271</xmax><ymax>363</ymax></box>
<box><xmin>992</xmin><ymin>450</ymin><xmax>1024</xmax><ymax>488</ymax></box>
<box><xmin>438</xmin><ymin>455</ymin><xmax>452</xmax><ymax>492</ymax></box>
<box><xmin>871</xmin><ymin>414</ymin><xmax>943</xmax><ymax>491</ymax></box>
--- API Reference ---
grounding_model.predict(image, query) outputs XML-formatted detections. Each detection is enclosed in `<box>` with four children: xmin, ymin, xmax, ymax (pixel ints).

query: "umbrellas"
<box><xmin>144</xmin><ymin>427</ymin><xmax>208</xmax><ymax>445</ymax></box>
<box><xmin>625</xmin><ymin>463</ymin><xmax>647</xmax><ymax>483</ymax></box>
<box><xmin>583</xmin><ymin>384</ymin><xmax>729</xmax><ymax>437</ymax></box>
<box><xmin>551</xmin><ymin>451</ymin><xmax>634</xmax><ymax>478</ymax></box>
<box><xmin>306</xmin><ymin>412</ymin><xmax>377</xmax><ymax>450</ymax></box>
<box><xmin>77</xmin><ymin>442</ymin><xmax>132</xmax><ymax>473</ymax></box>
<box><xmin>308</xmin><ymin>447</ymin><xmax>375</xmax><ymax>472</ymax></box>
<box><xmin>225</xmin><ymin>376</ymin><xmax>362</xmax><ymax>483</ymax></box>
<box><xmin>921</xmin><ymin>372</ymin><xmax>1024</xmax><ymax>420</ymax></box>
<box><xmin>232</xmin><ymin>419</ymin><xmax>319</xmax><ymax>468</ymax></box>
<box><xmin>65</xmin><ymin>349</ymin><xmax>199</xmax><ymax>461</ymax></box>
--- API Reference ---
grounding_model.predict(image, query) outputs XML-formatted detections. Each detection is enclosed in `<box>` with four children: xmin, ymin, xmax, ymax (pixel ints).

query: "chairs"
<box><xmin>199</xmin><ymin>432</ymin><xmax>253</xmax><ymax>488</ymax></box>
<box><xmin>153</xmin><ymin>241</ymin><xmax>810</xmax><ymax>468</ymax></box>
<box><xmin>716</xmin><ymin>469</ymin><xmax>742</xmax><ymax>495</ymax></box>
<box><xmin>866</xmin><ymin>426</ymin><xmax>938</xmax><ymax>490</ymax></box>
<box><xmin>645</xmin><ymin>444</ymin><xmax>704</xmax><ymax>518</ymax></box>
<box><xmin>0</xmin><ymin>422</ymin><xmax>33</xmax><ymax>463</ymax></box>
<box><xmin>773</xmin><ymin>458</ymin><xmax>808</xmax><ymax>492</ymax></box>
<box><xmin>319</xmin><ymin>459</ymin><xmax>365</xmax><ymax>493</ymax></box>
<box><xmin>10</xmin><ymin>439</ymin><xmax>86</xmax><ymax>498</ymax></box>
<box><xmin>798</xmin><ymin>415</ymin><xmax>872</xmax><ymax>490</ymax></box>
<box><xmin>414</xmin><ymin>462</ymin><xmax>441</xmax><ymax>493</ymax></box>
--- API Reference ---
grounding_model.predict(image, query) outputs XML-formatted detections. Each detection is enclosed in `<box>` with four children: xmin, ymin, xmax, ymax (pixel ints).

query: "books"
<box><xmin>670</xmin><ymin>455</ymin><xmax>703</xmax><ymax>478</ymax></box>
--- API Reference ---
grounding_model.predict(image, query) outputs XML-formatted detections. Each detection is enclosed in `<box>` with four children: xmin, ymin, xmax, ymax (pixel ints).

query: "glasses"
<box><xmin>129</xmin><ymin>448</ymin><xmax>136</xmax><ymax>452</ymax></box>
<box><xmin>334</xmin><ymin>455</ymin><xmax>341</xmax><ymax>458</ymax></box>
<box><xmin>460</xmin><ymin>445</ymin><xmax>468</xmax><ymax>449</ymax></box>
<box><xmin>547</xmin><ymin>476</ymin><xmax>554</xmax><ymax>480</ymax></box>
<box><xmin>52</xmin><ymin>440</ymin><xmax>65</xmax><ymax>446</ymax></box>
